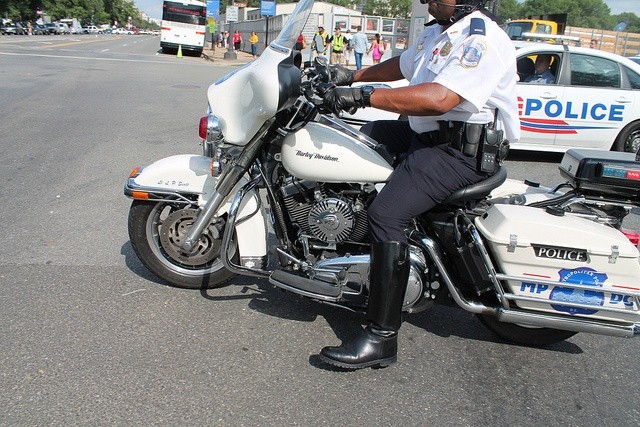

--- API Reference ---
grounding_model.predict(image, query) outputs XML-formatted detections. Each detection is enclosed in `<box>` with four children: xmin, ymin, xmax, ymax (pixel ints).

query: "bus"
<box><xmin>160</xmin><ymin>0</ymin><xmax>208</xmax><ymax>58</ymax></box>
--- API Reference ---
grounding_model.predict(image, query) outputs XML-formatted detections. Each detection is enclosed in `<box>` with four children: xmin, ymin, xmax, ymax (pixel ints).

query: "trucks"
<box><xmin>503</xmin><ymin>11</ymin><xmax>640</xmax><ymax>65</ymax></box>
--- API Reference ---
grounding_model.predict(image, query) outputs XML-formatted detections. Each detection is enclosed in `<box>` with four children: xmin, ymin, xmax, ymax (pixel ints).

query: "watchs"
<box><xmin>360</xmin><ymin>85</ymin><xmax>374</xmax><ymax>107</ymax></box>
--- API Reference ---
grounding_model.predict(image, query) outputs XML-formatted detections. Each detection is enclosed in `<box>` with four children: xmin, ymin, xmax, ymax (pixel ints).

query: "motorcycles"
<box><xmin>121</xmin><ymin>1</ymin><xmax>640</xmax><ymax>347</ymax></box>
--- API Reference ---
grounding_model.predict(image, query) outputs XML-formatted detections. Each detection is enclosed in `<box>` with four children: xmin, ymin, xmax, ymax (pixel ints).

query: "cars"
<box><xmin>122</xmin><ymin>25</ymin><xmax>159</xmax><ymax>36</ymax></box>
<box><xmin>2</xmin><ymin>17</ymin><xmax>121</xmax><ymax>37</ymax></box>
<box><xmin>334</xmin><ymin>40</ymin><xmax>640</xmax><ymax>157</ymax></box>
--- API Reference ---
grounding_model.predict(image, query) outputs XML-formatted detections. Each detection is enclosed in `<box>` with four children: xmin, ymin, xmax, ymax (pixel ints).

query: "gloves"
<box><xmin>323</xmin><ymin>87</ymin><xmax>365</xmax><ymax>119</ymax></box>
<box><xmin>329</xmin><ymin>63</ymin><xmax>355</xmax><ymax>86</ymax></box>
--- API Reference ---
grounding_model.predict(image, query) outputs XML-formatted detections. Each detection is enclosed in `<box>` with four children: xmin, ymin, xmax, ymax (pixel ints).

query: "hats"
<box><xmin>318</xmin><ymin>23</ymin><xmax>325</xmax><ymax>30</ymax></box>
<box><xmin>335</xmin><ymin>25</ymin><xmax>341</xmax><ymax>30</ymax></box>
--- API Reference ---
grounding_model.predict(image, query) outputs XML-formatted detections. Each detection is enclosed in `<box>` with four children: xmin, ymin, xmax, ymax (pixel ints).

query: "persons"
<box><xmin>328</xmin><ymin>27</ymin><xmax>348</xmax><ymax>66</ymax></box>
<box><xmin>590</xmin><ymin>39</ymin><xmax>597</xmax><ymax>49</ymax></box>
<box><xmin>319</xmin><ymin>0</ymin><xmax>522</xmax><ymax>371</ymax></box>
<box><xmin>349</xmin><ymin>26</ymin><xmax>369</xmax><ymax>70</ymax></box>
<box><xmin>231</xmin><ymin>28</ymin><xmax>242</xmax><ymax>51</ymax></box>
<box><xmin>218</xmin><ymin>29</ymin><xmax>231</xmax><ymax>49</ymax></box>
<box><xmin>310</xmin><ymin>27</ymin><xmax>329</xmax><ymax>56</ymax></box>
<box><xmin>248</xmin><ymin>31</ymin><xmax>259</xmax><ymax>60</ymax></box>
<box><xmin>524</xmin><ymin>54</ymin><xmax>555</xmax><ymax>84</ymax></box>
<box><xmin>342</xmin><ymin>30</ymin><xmax>353</xmax><ymax>67</ymax></box>
<box><xmin>367</xmin><ymin>33</ymin><xmax>386</xmax><ymax>66</ymax></box>
<box><xmin>290</xmin><ymin>28</ymin><xmax>306</xmax><ymax>51</ymax></box>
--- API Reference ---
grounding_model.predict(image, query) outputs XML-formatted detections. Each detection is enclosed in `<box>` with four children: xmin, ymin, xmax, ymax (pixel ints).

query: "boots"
<box><xmin>318</xmin><ymin>239</ymin><xmax>410</xmax><ymax>370</ymax></box>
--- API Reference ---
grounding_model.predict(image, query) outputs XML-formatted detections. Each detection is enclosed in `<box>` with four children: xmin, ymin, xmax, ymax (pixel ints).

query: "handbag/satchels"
<box><xmin>379</xmin><ymin>41</ymin><xmax>385</xmax><ymax>55</ymax></box>
<box><xmin>302</xmin><ymin>41</ymin><xmax>307</xmax><ymax>49</ymax></box>
